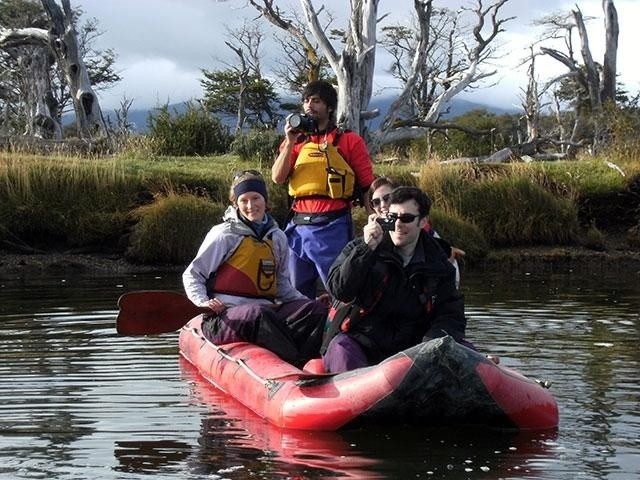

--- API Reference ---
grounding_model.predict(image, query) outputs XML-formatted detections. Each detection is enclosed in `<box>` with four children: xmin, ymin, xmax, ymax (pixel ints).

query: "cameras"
<box><xmin>376</xmin><ymin>218</ymin><xmax>395</xmax><ymax>231</ymax></box>
<box><xmin>288</xmin><ymin>112</ymin><xmax>317</xmax><ymax>133</ymax></box>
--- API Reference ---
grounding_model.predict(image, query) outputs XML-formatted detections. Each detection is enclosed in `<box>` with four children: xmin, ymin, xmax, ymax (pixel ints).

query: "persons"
<box><xmin>320</xmin><ymin>184</ymin><xmax>474</xmax><ymax>376</ymax></box>
<box><xmin>369</xmin><ymin>178</ymin><xmax>468</xmax><ymax>289</ymax></box>
<box><xmin>182</xmin><ymin>168</ymin><xmax>327</xmax><ymax>368</ymax></box>
<box><xmin>273</xmin><ymin>80</ymin><xmax>375</xmax><ymax>299</ymax></box>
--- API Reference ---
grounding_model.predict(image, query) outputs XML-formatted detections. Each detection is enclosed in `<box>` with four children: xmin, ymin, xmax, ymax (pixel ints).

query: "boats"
<box><xmin>179</xmin><ymin>312</ymin><xmax>559</xmax><ymax>430</ymax></box>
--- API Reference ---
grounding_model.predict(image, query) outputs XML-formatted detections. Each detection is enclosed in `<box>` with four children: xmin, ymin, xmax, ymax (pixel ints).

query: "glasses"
<box><xmin>369</xmin><ymin>193</ymin><xmax>393</xmax><ymax>207</ymax></box>
<box><xmin>385</xmin><ymin>211</ymin><xmax>421</xmax><ymax>224</ymax></box>
<box><xmin>233</xmin><ymin>169</ymin><xmax>260</xmax><ymax>181</ymax></box>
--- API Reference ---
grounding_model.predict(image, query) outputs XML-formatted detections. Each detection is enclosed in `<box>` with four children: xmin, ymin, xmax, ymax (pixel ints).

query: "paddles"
<box><xmin>116</xmin><ymin>290</ymin><xmax>215</xmax><ymax>336</ymax></box>
<box><xmin>267</xmin><ymin>372</ymin><xmax>340</xmax><ymax>381</ymax></box>
